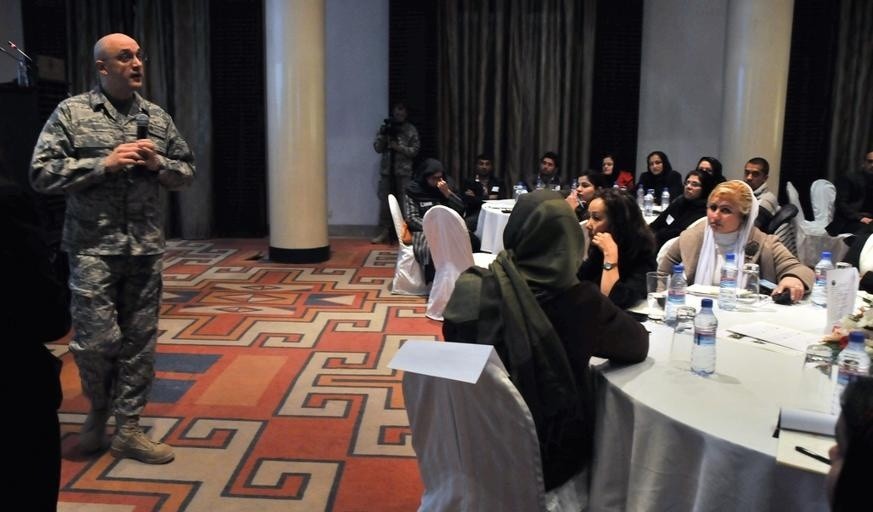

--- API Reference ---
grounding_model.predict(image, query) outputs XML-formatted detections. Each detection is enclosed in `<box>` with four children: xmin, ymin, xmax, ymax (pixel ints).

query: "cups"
<box><xmin>669</xmin><ymin>305</ymin><xmax>694</xmax><ymax>366</ymax></box>
<box><xmin>800</xmin><ymin>343</ymin><xmax>833</xmax><ymax>413</ymax></box>
<box><xmin>740</xmin><ymin>262</ymin><xmax>760</xmax><ymax>308</ymax></box>
<box><xmin>646</xmin><ymin>271</ymin><xmax>669</xmax><ymax>321</ymax></box>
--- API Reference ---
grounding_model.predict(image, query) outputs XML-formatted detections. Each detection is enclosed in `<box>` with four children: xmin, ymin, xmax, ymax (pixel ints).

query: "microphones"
<box><xmin>7</xmin><ymin>41</ymin><xmax>32</xmax><ymax>61</ymax></box>
<box><xmin>133</xmin><ymin>114</ymin><xmax>149</xmax><ymax>178</ymax></box>
<box><xmin>0</xmin><ymin>47</ymin><xmax>29</xmax><ymax>65</ymax></box>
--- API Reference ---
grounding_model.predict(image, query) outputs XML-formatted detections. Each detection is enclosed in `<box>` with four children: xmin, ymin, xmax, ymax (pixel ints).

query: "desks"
<box><xmin>480</xmin><ymin>197</ymin><xmax>669</xmax><ymax>254</ymax></box>
<box><xmin>586</xmin><ymin>272</ymin><xmax>871</xmax><ymax>512</ymax></box>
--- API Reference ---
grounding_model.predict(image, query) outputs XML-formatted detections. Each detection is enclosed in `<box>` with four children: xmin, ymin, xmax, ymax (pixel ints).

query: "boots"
<box><xmin>112</xmin><ymin>413</ymin><xmax>175</xmax><ymax>464</ymax></box>
<box><xmin>79</xmin><ymin>410</ymin><xmax>113</xmax><ymax>451</ymax></box>
<box><xmin>371</xmin><ymin>229</ymin><xmax>389</xmax><ymax>244</ymax></box>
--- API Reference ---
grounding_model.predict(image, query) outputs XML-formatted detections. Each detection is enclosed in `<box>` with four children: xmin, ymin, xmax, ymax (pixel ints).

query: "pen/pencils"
<box><xmin>795</xmin><ymin>445</ymin><xmax>830</xmax><ymax>466</ymax></box>
<box><xmin>576</xmin><ymin>196</ymin><xmax>585</xmax><ymax>208</ymax></box>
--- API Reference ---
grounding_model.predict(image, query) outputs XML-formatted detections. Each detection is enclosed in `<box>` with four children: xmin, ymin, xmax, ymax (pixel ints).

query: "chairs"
<box><xmin>422</xmin><ymin>205</ymin><xmax>505</xmax><ymax>322</ymax></box>
<box><xmin>401</xmin><ymin>353</ymin><xmax>586</xmax><ymax>512</ymax></box>
<box><xmin>809</xmin><ymin>179</ymin><xmax>837</xmax><ymax>221</ymax></box>
<box><xmin>387</xmin><ymin>193</ymin><xmax>438</xmax><ymax>295</ymax></box>
<box><xmin>785</xmin><ymin>180</ymin><xmax>830</xmax><ymax>267</ymax></box>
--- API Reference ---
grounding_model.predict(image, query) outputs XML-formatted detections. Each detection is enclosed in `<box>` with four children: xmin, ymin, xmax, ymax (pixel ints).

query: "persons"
<box><xmin>828</xmin><ymin>147</ymin><xmax>873</xmax><ymax>269</ymax></box>
<box><xmin>440</xmin><ymin>186</ymin><xmax>649</xmax><ymax>494</ymax></box>
<box><xmin>27</xmin><ymin>32</ymin><xmax>197</xmax><ymax>467</ymax></box>
<box><xmin>826</xmin><ymin>369</ymin><xmax>873</xmax><ymax>511</ymax></box>
<box><xmin>370</xmin><ymin>102</ymin><xmax>420</xmax><ymax>244</ymax></box>
<box><xmin>402</xmin><ymin>150</ymin><xmax>781</xmax><ymax>286</ymax></box>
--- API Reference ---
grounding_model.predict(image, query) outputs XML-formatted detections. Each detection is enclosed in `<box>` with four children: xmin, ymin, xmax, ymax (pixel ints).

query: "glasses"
<box><xmin>684</xmin><ymin>181</ymin><xmax>701</xmax><ymax>186</ymax></box>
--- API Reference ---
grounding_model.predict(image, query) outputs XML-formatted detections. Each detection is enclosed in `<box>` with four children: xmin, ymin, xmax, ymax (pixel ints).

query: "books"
<box><xmin>772</xmin><ymin>403</ymin><xmax>843</xmax><ymax>479</ymax></box>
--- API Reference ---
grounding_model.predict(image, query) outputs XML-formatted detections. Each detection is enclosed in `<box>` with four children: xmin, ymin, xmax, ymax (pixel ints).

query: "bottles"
<box><xmin>660</xmin><ymin>187</ymin><xmax>669</xmax><ymax>210</ymax></box>
<box><xmin>512</xmin><ymin>175</ymin><xmax>576</xmax><ymax>203</ymax></box>
<box><xmin>692</xmin><ymin>298</ymin><xmax>716</xmax><ymax>373</ymax></box>
<box><xmin>644</xmin><ymin>190</ymin><xmax>653</xmax><ymax>216</ymax></box>
<box><xmin>813</xmin><ymin>251</ymin><xmax>833</xmax><ymax>305</ymax></box>
<box><xmin>716</xmin><ymin>254</ymin><xmax>738</xmax><ymax>312</ymax></box>
<box><xmin>667</xmin><ymin>266</ymin><xmax>686</xmax><ymax>321</ymax></box>
<box><xmin>636</xmin><ymin>184</ymin><xmax>644</xmax><ymax>207</ymax></box>
<box><xmin>834</xmin><ymin>332</ymin><xmax>870</xmax><ymax>408</ymax></box>
